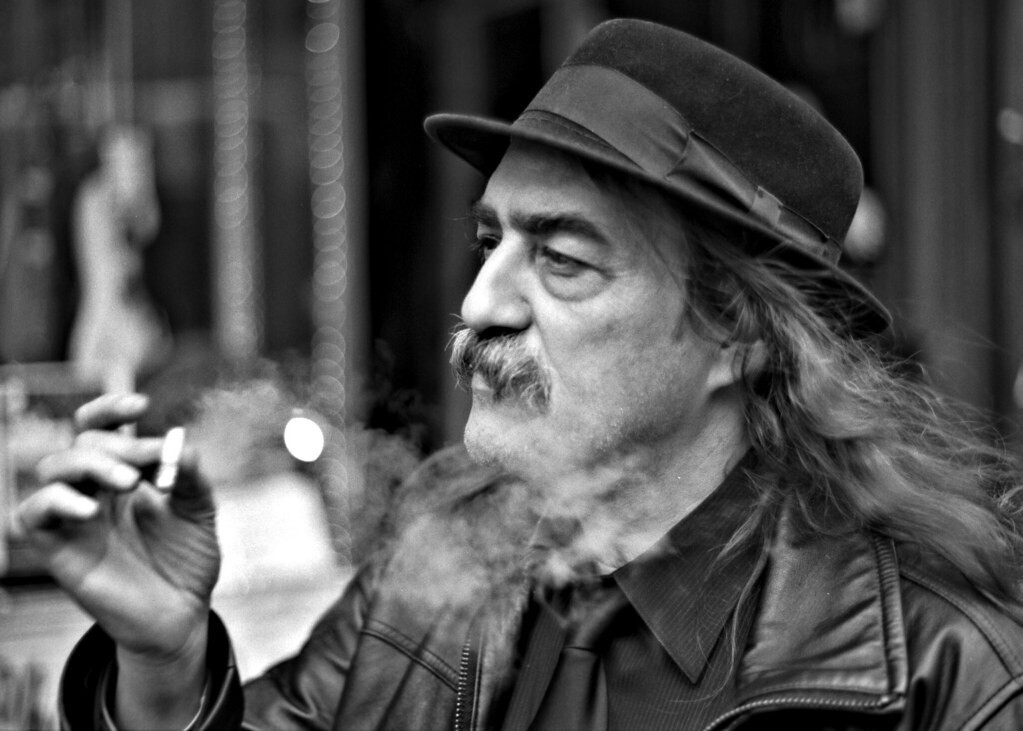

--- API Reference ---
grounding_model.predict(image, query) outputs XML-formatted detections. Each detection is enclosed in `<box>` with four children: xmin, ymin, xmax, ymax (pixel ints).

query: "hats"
<box><xmin>423</xmin><ymin>20</ymin><xmax>892</xmax><ymax>338</ymax></box>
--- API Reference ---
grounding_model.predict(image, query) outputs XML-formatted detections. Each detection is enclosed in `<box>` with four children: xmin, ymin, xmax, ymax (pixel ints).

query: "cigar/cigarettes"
<box><xmin>157</xmin><ymin>428</ymin><xmax>185</xmax><ymax>487</ymax></box>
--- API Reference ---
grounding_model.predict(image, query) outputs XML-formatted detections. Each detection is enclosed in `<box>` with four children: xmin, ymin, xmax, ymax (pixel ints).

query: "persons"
<box><xmin>17</xmin><ymin>18</ymin><xmax>1023</xmax><ymax>731</ymax></box>
<box><xmin>71</xmin><ymin>130</ymin><xmax>168</xmax><ymax>431</ymax></box>
